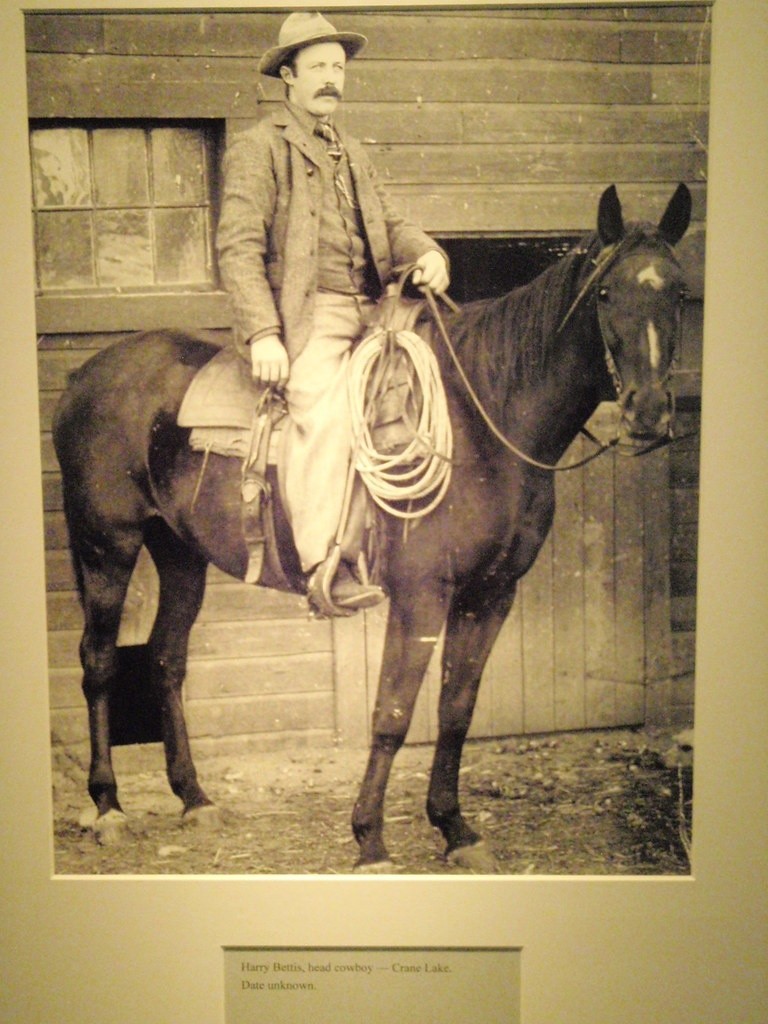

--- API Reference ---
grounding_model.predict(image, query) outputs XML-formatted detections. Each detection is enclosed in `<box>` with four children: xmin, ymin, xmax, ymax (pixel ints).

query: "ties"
<box><xmin>313</xmin><ymin>120</ymin><xmax>342</xmax><ymax>165</ymax></box>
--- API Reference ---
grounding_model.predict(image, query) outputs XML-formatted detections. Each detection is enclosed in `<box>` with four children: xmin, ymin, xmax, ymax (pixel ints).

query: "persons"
<box><xmin>214</xmin><ymin>10</ymin><xmax>451</xmax><ymax>620</ymax></box>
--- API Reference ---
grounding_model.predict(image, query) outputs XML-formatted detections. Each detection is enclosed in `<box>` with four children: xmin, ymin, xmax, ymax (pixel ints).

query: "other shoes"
<box><xmin>307</xmin><ymin>560</ymin><xmax>385</xmax><ymax>620</ymax></box>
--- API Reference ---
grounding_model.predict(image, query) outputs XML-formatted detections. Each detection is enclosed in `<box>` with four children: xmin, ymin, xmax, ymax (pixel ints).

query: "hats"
<box><xmin>258</xmin><ymin>11</ymin><xmax>367</xmax><ymax>79</ymax></box>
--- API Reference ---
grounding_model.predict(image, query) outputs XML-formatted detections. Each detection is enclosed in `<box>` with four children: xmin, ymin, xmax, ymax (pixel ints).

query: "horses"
<box><xmin>52</xmin><ymin>181</ymin><xmax>692</xmax><ymax>874</ymax></box>
